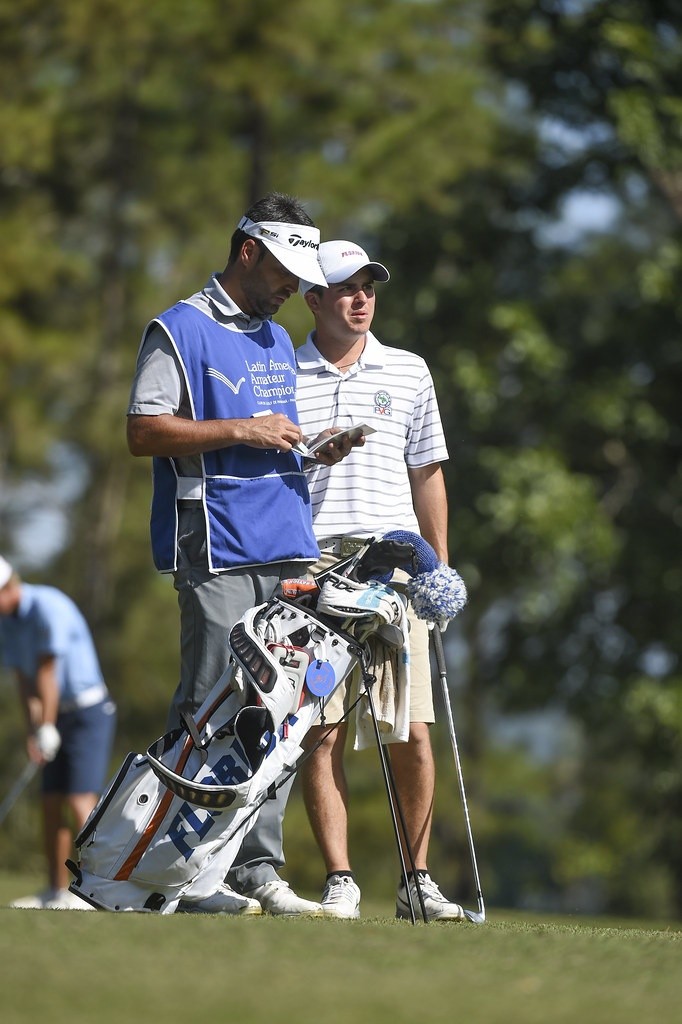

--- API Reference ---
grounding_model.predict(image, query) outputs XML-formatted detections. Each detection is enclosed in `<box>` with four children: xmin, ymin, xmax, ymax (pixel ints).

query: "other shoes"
<box><xmin>9</xmin><ymin>889</ymin><xmax>97</xmax><ymax>910</ymax></box>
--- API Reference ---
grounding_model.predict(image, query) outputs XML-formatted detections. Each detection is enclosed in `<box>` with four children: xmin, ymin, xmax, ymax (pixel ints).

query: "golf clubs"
<box><xmin>1</xmin><ymin>761</ymin><xmax>36</xmax><ymax>820</ymax></box>
<box><xmin>354</xmin><ymin>613</ymin><xmax>406</xmax><ymax>650</ymax></box>
<box><xmin>431</xmin><ymin>622</ymin><xmax>488</xmax><ymax>925</ymax></box>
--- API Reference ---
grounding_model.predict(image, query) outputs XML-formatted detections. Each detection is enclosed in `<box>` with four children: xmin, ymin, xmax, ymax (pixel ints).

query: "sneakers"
<box><xmin>177</xmin><ymin>881</ymin><xmax>261</xmax><ymax>914</ymax></box>
<box><xmin>240</xmin><ymin>881</ymin><xmax>324</xmax><ymax>916</ymax></box>
<box><xmin>396</xmin><ymin>872</ymin><xmax>465</xmax><ymax>919</ymax></box>
<box><xmin>320</xmin><ymin>875</ymin><xmax>361</xmax><ymax>918</ymax></box>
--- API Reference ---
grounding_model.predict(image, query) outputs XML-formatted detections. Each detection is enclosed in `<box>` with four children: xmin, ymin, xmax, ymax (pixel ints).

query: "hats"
<box><xmin>237</xmin><ymin>216</ymin><xmax>329</xmax><ymax>289</ymax></box>
<box><xmin>299</xmin><ymin>240</ymin><xmax>391</xmax><ymax>299</ymax></box>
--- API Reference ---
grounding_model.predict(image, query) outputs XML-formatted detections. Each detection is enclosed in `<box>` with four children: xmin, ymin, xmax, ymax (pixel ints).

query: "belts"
<box><xmin>318</xmin><ymin>538</ymin><xmax>361</xmax><ymax>557</ymax></box>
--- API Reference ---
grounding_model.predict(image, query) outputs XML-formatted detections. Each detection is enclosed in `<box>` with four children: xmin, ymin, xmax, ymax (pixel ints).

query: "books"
<box><xmin>251</xmin><ymin>409</ymin><xmax>376</xmax><ymax>459</ymax></box>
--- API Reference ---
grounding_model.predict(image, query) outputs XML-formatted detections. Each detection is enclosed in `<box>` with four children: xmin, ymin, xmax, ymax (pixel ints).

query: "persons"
<box><xmin>1</xmin><ymin>557</ymin><xmax>116</xmax><ymax>910</ymax></box>
<box><xmin>125</xmin><ymin>188</ymin><xmax>366</xmax><ymax>915</ymax></box>
<box><xmin>295</xmin><ymin>238</ymin><xmax>464</xmax><ymax>922</ymax></box>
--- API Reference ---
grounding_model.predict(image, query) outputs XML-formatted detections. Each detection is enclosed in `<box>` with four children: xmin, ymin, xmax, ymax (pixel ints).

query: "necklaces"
<box><xmin>337</xmin><ymin>362</ymin><xmax>355</xmax><ymax>369</ymax></box>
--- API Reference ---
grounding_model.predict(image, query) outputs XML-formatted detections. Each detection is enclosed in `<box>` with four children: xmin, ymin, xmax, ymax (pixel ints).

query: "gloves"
<box><xmin>35</xmin><ymin>724</ymin><xmax>60</xmax><ymax>761</ymax></box>
